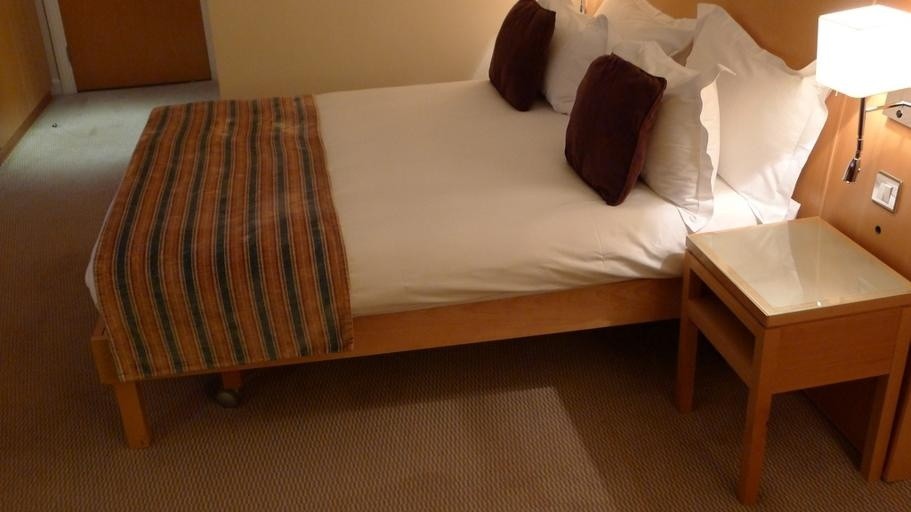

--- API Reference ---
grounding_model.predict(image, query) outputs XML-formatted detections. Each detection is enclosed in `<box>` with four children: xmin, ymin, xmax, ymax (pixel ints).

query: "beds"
<box><xmin>79</xmin><ymin>0</ymin><xmax>849</xmax><ymax>450</ymax></box>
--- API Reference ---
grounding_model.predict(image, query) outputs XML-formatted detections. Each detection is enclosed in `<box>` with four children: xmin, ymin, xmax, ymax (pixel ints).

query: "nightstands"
<box><xmin>676</xmin><ymin>224</ymin><xmax>909</xmax><ymax>507</ymax></box>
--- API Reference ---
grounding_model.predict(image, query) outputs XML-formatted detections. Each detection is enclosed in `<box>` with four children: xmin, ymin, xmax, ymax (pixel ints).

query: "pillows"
<box><xmin>676</xmin><ymin>4</ymin><xmax>836</xmax><ymax>226</ymax></box>
<box><xmin>563</xmin><ymin>52</ymin><xmax>668</xmax><ymax>205</ymax></box>
<box><xmin>485</xmin><ymin>1</ymin><xmax>557</xmax><ymax>113</ymax></box>
<box><xmin>536</xmin><ymin>2</ymin><xmax>615</xmax><ymax>112</ymax></box>
<box><xmin>621</xmin><ymin>38</ymin><xmax>723</xmax><ymax>229</ymax></box>
<box><xmin>590</xmin><ymin>0</ymin><xmax>705</xmax><ymax>100</ymax></box>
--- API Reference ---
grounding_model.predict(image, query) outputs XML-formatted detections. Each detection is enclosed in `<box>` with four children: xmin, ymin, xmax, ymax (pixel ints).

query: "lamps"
<box><xmin>815</xmin><ymin>4</ymin><xmax>911</xmax><ymax>187</ymax></box>
